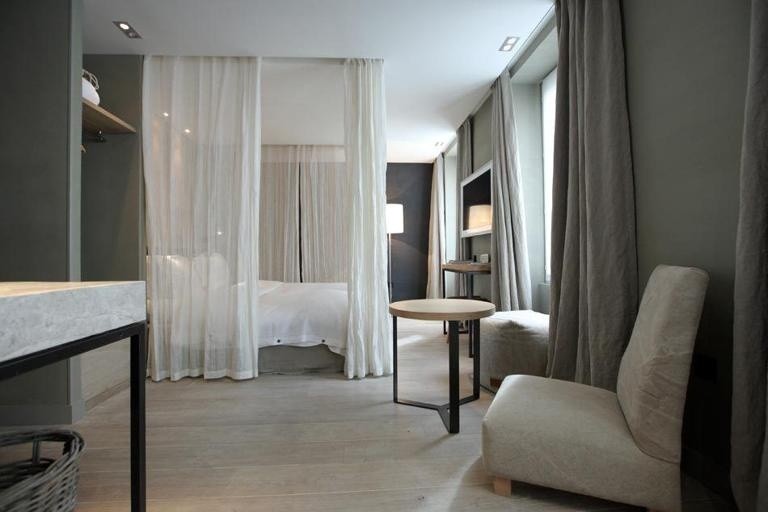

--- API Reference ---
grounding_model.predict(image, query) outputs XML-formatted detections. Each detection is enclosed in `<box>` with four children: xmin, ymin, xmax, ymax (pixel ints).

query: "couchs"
<box><xmin>479</xmin><ymin>306</ymin><xmax>551</xmax><ymax>394</ymax></box>
<box><xmin>482</xmin><ymin>261</ymin><xmax>711</xmax><ymax>512</ymax></box>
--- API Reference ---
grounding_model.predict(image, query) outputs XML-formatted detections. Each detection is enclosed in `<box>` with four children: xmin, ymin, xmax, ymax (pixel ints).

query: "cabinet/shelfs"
<box><xmin>440</xmin><ymin>159</ymin><xmax>496</xmax><ymax>333</ymax></box>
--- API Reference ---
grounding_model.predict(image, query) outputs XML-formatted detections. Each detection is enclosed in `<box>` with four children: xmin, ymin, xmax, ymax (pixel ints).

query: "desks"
<box><xmin>0</xmin><ymin>280</ymin><xmax>151</xmax><ymax>512</ymax></box>
<box><xmin>388</xmin><ymin>297</ymin><xmax>497</xmax><ymax>434</ymax></box>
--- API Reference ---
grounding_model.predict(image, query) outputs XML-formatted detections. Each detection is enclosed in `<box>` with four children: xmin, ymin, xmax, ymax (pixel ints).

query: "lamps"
<box><xmin>385</xmin><ymin>203</ymin><xmax>405</xmax><ymax>303</ymax></box>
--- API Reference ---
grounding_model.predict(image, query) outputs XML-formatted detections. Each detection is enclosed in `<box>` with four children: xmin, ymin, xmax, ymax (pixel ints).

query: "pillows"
<box><xmin>146</xmin><ymin>254</ymin><xmax>201</xmax><ymax>294</ymax></box>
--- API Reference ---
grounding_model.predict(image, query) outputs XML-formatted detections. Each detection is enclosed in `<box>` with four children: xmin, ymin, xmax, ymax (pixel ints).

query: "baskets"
<box><xmin>0</xmin><ymin>425</ymin><xmax>86</xmax><ymax>511</ymax></box>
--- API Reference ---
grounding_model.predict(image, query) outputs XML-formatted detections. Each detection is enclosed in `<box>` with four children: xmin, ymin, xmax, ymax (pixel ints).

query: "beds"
<box><xmin>145</xmin><ymin>245</ymin><xmax>385</xmax><ymax>377</ymax></box>
